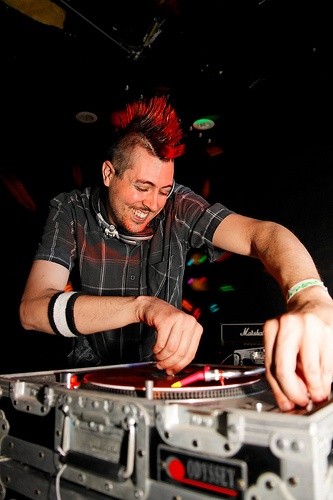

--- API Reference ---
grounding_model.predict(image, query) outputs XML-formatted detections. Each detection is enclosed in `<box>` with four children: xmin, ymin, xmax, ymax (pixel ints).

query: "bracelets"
<box><xmin>48</xmin><ymin>289</ymin><xmax>86</xmax><ymax>339</ymax></box>
<box><xmin>286</xmin><ymin>278</ymin><xmax>328</xmax><ymax>304</ymax></box>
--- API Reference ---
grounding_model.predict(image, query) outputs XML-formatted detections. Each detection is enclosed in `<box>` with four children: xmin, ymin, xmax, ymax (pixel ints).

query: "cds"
<box><xmin>85</xmin><ymin>364</ymin><xmax>261</xmax><ymax>391</ymax></box>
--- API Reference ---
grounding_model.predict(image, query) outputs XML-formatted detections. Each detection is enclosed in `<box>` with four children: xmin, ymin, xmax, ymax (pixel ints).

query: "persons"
<box><xmin>18</xmin><ymin>93</ymin><xmax>332</xmax><ymax>411</ymax></box>
